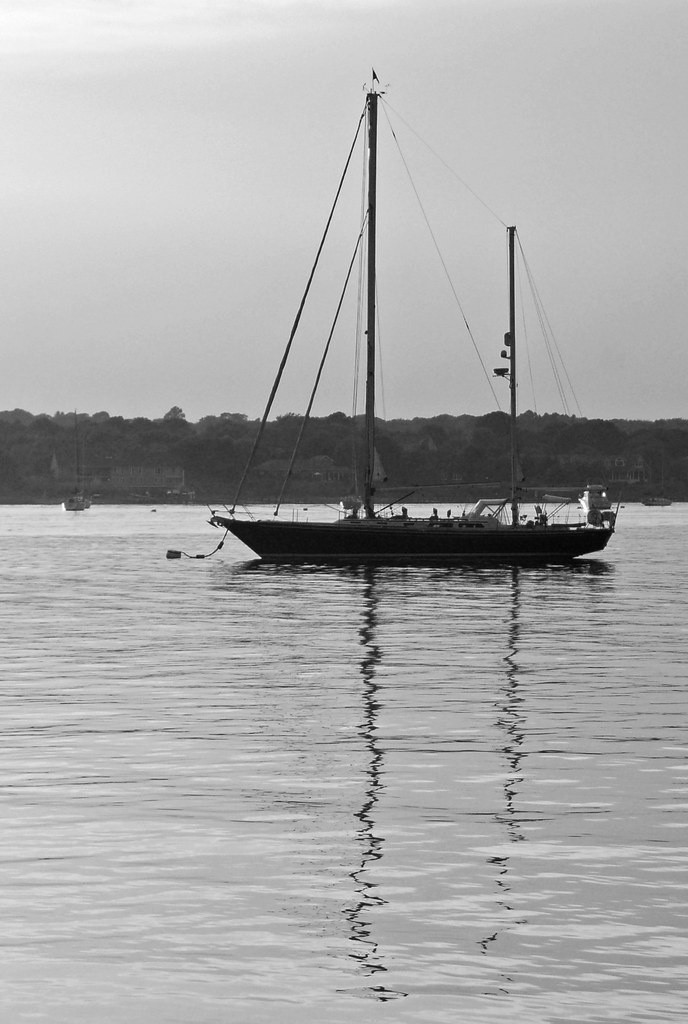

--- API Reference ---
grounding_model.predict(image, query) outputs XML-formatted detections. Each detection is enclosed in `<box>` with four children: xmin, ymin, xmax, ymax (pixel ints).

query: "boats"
<box><xmin>645</xmin><ymin>497</ymin><xmax>672</xmax><ymax>506</ymax></box>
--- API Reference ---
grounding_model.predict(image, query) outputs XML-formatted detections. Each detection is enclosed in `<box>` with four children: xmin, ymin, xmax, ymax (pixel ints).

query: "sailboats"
<box><xmin>210</xmin><ymin>65</ymin><xmax>624</xmax><ymax>568</ymax></box>
<box><xmin>65</xmin><ymin>409</ymin><xmax>92</xmax><ymax>511</ymax></box>
